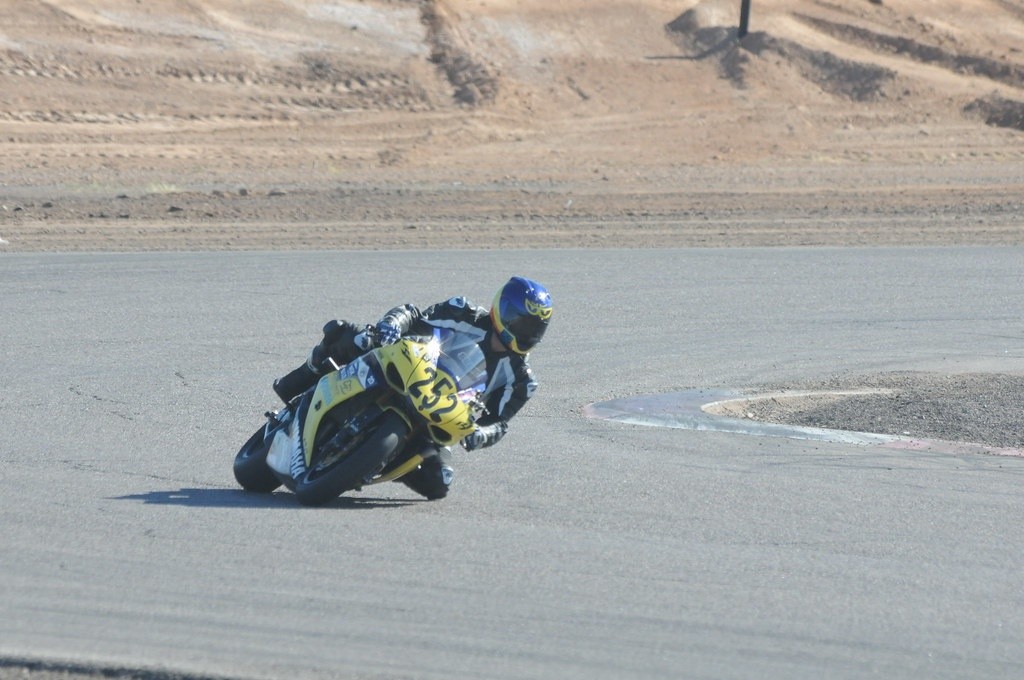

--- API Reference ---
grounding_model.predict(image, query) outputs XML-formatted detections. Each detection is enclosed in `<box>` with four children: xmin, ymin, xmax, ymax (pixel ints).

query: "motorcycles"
<box><xmin>233</xmin><ymin>324</ymin><xmax>490</xmax><ymax>508</ymax></box>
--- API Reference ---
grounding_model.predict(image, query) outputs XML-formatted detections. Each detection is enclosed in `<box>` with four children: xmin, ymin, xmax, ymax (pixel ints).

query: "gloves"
<box><xmin>375</xmin><ymin>320</ymin><xmax>400</xmax><ymax>346</ymax></box>
<box><xmin>461</xmin><ymin>425</ymin><xmax>484</xmax><ymax>451</ymax></box>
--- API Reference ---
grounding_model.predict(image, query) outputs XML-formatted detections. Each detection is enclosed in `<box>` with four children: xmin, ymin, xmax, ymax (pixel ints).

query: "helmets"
<box><xmin>490</xmin><ymin>277</ymin><xmax>553</xmax><ymax>355</ymax></box>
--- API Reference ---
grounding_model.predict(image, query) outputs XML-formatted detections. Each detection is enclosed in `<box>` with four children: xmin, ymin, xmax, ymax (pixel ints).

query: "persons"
<box><xmin>276</xmin><ymin>277</ymin><xmax>552</xmax><ymax>502</ymax></box>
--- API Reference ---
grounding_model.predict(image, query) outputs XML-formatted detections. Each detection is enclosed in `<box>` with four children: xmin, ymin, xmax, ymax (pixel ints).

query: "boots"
<box><xmin>278</xmin><ymin>346</ymin><xmax>322</xmax><ymax>398</ymax></box>
